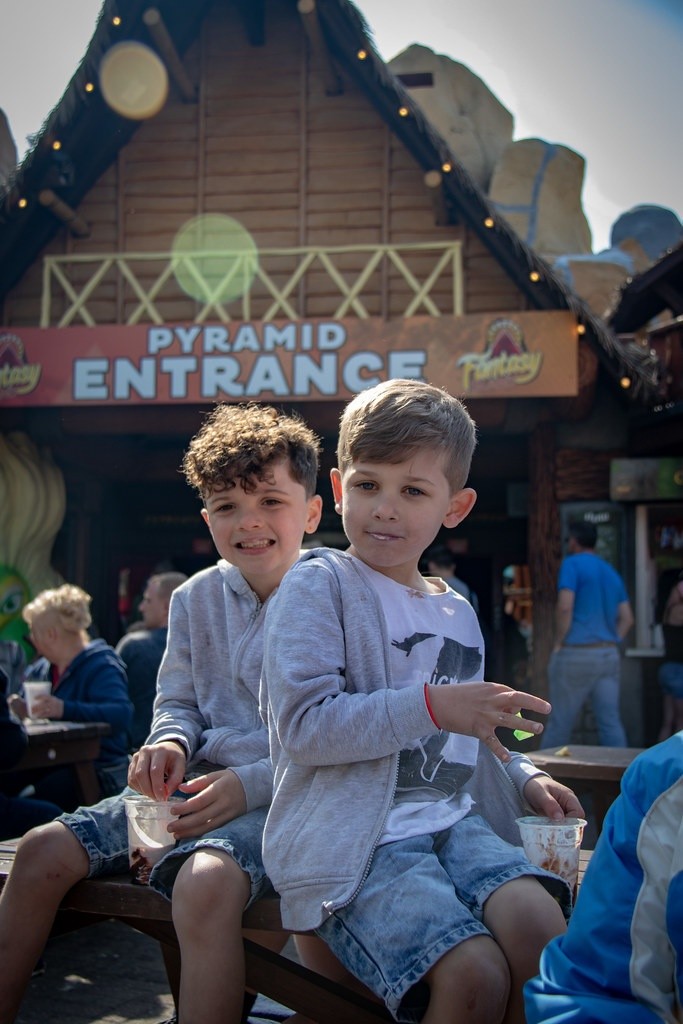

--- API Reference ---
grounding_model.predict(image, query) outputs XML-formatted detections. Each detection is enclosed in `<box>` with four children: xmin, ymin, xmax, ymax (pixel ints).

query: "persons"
<box><xmin>658</xmin><ymin>569</ymin><xmax>683</xmax><ymax>745</ymax></box>
<box><xmin>0</xmin><ymin>405</ymin><xmax>322</xmax><ymax>1024</ymax></box>
<box><xmin>0</xmin><ymin>666</ymin><xmax>65</xmax><ymax>844</ymax></box>
<box><xmin>114</xmin><ymin>573</ymin><xmax>188</xmax><ymax>752</ymax></box>
<box><xmin>428</xmin><ymin>543</ymin><xmax>479</xmax><ymax>618</ymax></box>
<box><xmin>258</xmin><ymin>378</ymin><xmax>572</xmax><ymax>1024</ymax></box>
<box><xmin>7</xmin><ymin>583</ymin><xmax>134</xmax><ymax>814</ymax></box>
<box><xmin>524</xmin><ymin>731</ymin><xmax>683</xmax><ymax>1024</ymax></box>
<box><xmin>539</xmin><ymin>521</ymin><xmax>633</xmax><ymax>750</ymax></box>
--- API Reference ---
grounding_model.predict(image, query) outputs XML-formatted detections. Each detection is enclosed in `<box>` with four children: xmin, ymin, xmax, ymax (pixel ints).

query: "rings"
<box><xmin>46</xmin><ymin>708</ymin><xmax>50</xmax><ymax>713</ymax></box>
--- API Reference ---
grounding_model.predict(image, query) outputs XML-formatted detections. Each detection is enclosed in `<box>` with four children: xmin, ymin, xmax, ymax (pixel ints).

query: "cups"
<box><xmin>23</xmin><ymin>681</ymin><xmax>51</xmax><ymax>719</ymax></box>
<box><xmin>120</xmin><ymin>795</ymin><xmax>186</xmax><ymax>886</ymax></box>
<box><xmin>515</xmin><ymin>816</ymin><xmax>588</xmax><ymax>908</ymax></box>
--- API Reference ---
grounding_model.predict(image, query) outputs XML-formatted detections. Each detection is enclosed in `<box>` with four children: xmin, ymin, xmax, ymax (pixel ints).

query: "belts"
<box><xmin>561</xmin><ymin>641</ymin><xmax>618</xmax><ymax>648</ymax></box>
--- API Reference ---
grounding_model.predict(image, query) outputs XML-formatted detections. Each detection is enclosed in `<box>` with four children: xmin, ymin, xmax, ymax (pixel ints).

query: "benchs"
<box><xmin>0</xmin><ymin>836</ymin><xmax>593</xmax><ymax>1024</ymax></box>
<box><xmin>523</xmin><ymin>743</ymin><xmax>647</xmax><ymax>833</ymax></box>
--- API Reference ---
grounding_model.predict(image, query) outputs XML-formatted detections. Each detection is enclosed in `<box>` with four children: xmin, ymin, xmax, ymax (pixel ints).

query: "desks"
<box><xmin>18</xmin><ymin>719</ymin><xmax>110</xmax><ymax>806</ymax></box>
